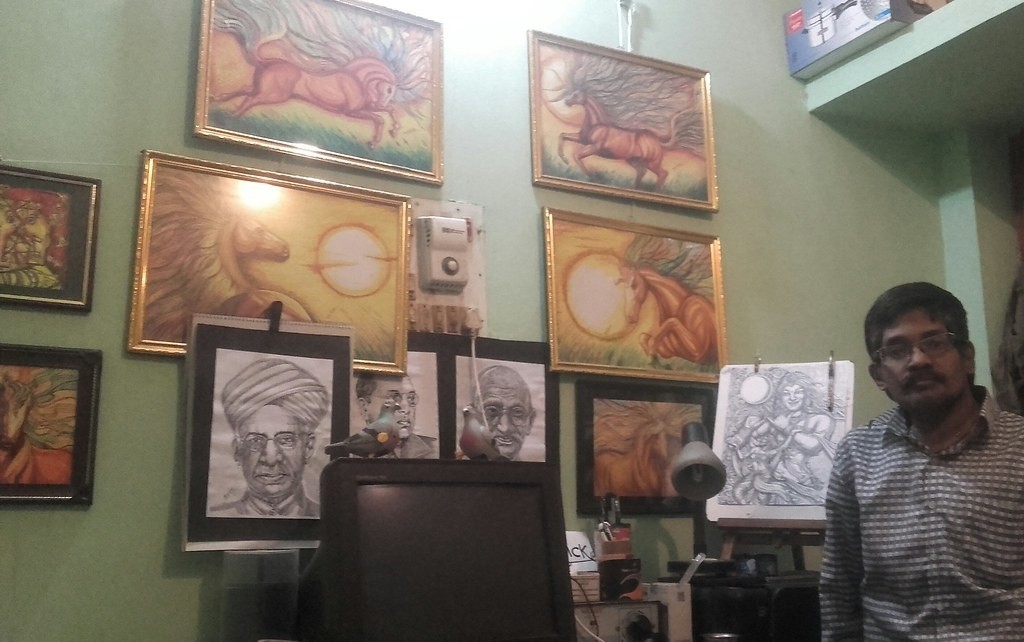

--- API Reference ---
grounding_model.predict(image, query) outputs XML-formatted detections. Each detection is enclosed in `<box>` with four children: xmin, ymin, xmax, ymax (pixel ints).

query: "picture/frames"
<box><xmin>0</xmin><ymin>163</ymin><xmax>103</xmax><ymax>314</ymax></box>
<box><xmin>189</xmin><ymin>0</ymin><xmax>446</xmax><ymax>187</ymax></box>
<box><xmin>541</xmin><ymin>206</ymin><xmax>730</xmax><ymax>383</ymax></box>
<box><xmin>574</xmin><ymin>378</ymin><xmax>717</xmax><ymax>517</ymax></box>
<box><xmin>123</xmin><ymin>147</ymin><xmax>413</xmax><ymax>374</ymax></box>
<box><xmin>180</xmin><ymin>313</ymin><xmax>357</xmax><ymax>551</ymax></box>
<box><xmin>526</xmin><ymin>29</ymin><xmax>720</xmax><ymax>214</ymax></box>
<box><xmin>0</xmin><ymin>342</ymin><xmax>104</xmax><ymax>508</ymax></box>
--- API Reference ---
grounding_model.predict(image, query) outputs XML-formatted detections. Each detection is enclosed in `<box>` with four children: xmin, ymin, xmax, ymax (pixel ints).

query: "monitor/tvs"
<box><xmin>298</xmin><ymin>457</ymin><xmax>577</xmax><ymax>642</ymax></box>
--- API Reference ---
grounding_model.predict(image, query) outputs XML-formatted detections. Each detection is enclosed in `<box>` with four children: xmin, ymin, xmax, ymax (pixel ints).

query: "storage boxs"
<box><xmin>782</xmin><ymin>0</ymin><xmax>913</xmax><ymax>80</ymax></box>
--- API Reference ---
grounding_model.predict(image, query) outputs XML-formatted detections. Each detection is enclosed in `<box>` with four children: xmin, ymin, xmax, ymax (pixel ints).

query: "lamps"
<box><xmin>666</xmin><ymin>421</ymin><xmax>728</xmax><ymax>574</ymax></box>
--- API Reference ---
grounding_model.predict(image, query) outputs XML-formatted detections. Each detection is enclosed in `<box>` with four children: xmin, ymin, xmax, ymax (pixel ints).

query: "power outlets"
<box><xmin>410</xmin><ymin>304</ymin><xmax>482</xmax><ymax>336</ymax></box>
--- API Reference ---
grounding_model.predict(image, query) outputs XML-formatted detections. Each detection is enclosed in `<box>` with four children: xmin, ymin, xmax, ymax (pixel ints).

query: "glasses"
<box><xmin>871</xmin><ymin>332</ymin><xmax>960</xmax><ymax>366</ymax></box>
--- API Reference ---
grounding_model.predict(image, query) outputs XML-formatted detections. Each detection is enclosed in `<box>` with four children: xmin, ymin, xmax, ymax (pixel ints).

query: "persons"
<box><xmin>819</xmin><ymin>282</ymin><xmax>1024</xmax><ymax>642</ymax></box>
